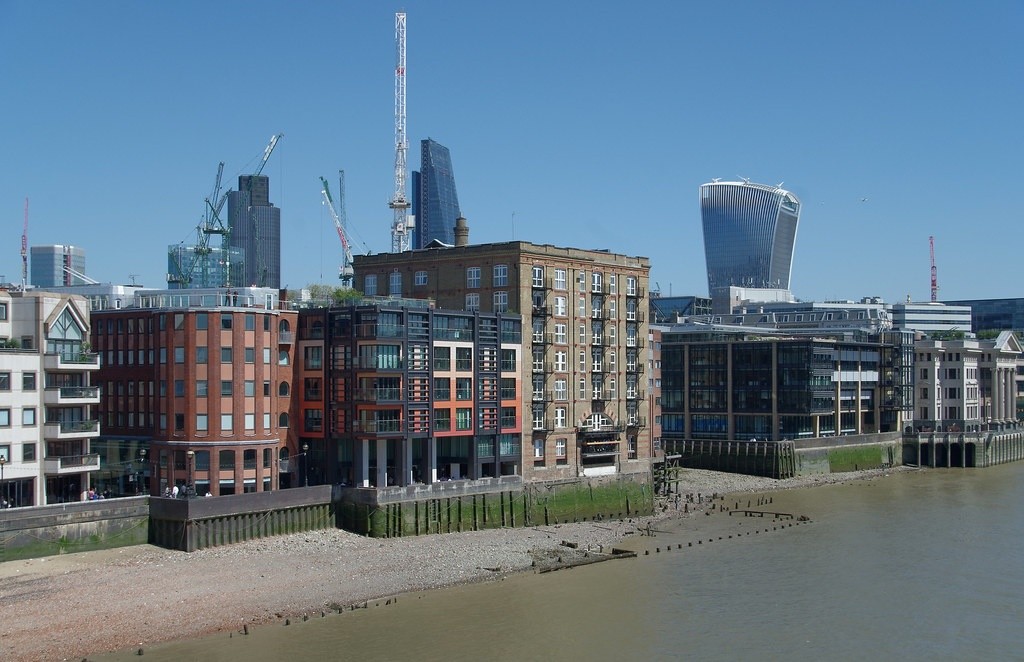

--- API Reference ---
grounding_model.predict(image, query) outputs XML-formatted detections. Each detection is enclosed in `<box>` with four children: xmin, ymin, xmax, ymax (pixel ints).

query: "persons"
<box><xmin>205</xmin><ymin>491</ymin><xmax>212</xmax><ymax>497</ymax></box>
<box><xmin>164</xmin><ymin>483</ymin><xmax>188</xmax><ymax>497</ymax></box>
<box><xmin>0</xmin><ymin>496</ymin><xmax>16</xmax><ymax>508</ymax></box>
<box><xmin>79</xmin><ymin>488</ymin><xmax>113</xmax><ymax>500</ymax></box>
